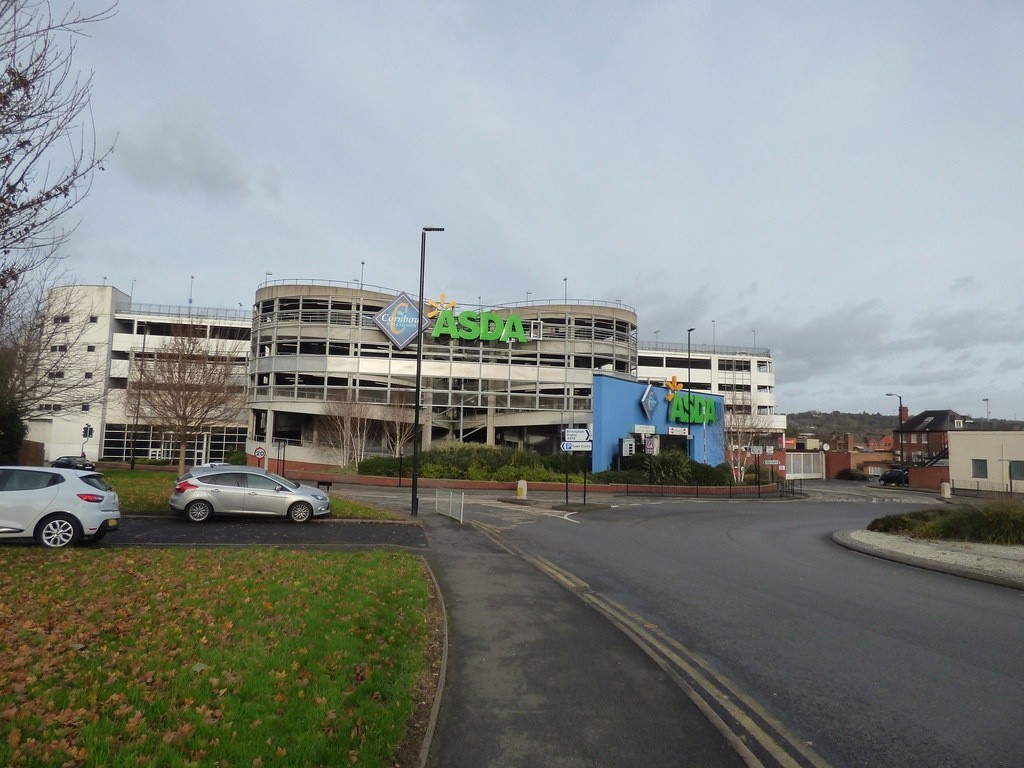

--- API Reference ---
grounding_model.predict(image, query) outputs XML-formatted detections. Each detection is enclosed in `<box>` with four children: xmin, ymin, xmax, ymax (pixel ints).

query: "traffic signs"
<box><xmin>560</xmin><ymin>441</ymin><xmax>593</xmax><ymax>452</ymax></box>
<box><xmin>565</xmin><ymin>429</ymin><xmax>591</xmax><ymax>442</ymax></box>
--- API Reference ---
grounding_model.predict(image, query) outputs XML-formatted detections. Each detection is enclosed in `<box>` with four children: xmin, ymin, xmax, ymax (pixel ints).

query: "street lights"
<box><xmin>884</xmin><ymin>393</ymin><xmax>906</xmax><ymax>487</ymax></box>
<box><xmin>686</xmin><ymin>328</ymin><xmax>696</xmax><ymax>457</ymax></box>
<box><xmin>129</xmin><ymin>320</ymin><xmax>149</xmax><ymax>471</ymax></box>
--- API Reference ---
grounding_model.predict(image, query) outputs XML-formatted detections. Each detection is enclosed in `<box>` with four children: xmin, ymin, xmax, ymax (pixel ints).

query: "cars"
<box><xmin>0</xmin><ymin>465</ymin><xmax>121</xmax><ymax>549</ymax></box>
<box><xmin>879</xmin><ymin>469</ymin><xmax>910</xmax><ymax>486</ymax></box>
<box><xmin>169</xmin><ymin>466</ymin><xmax>331</xmax><ymax>524</ymax></box>
<box><xmin>52</xmin><ymin>455</ymin><xmax>97</xmax><ymax>471</ymax></box>
<box><xmin>983</xmin><ymin>397</ymin><xmax>990</xmax><ymax>430</ymax></box>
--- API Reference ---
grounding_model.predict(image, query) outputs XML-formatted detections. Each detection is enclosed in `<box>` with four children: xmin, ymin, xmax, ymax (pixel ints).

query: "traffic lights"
<box><xmin>82</xmin><ymin>427</ymin><xmax>89</xmax><ymax>439</ymax></box>
<box><xmin>411</xmin><ymin>227</ymin><xmax>446</xmax><ymax>516</ymax></box>
<box><xmin>89</xmin><ymin>428</ymin><xmax>94</xmax><ymax>438</ymax></box>
